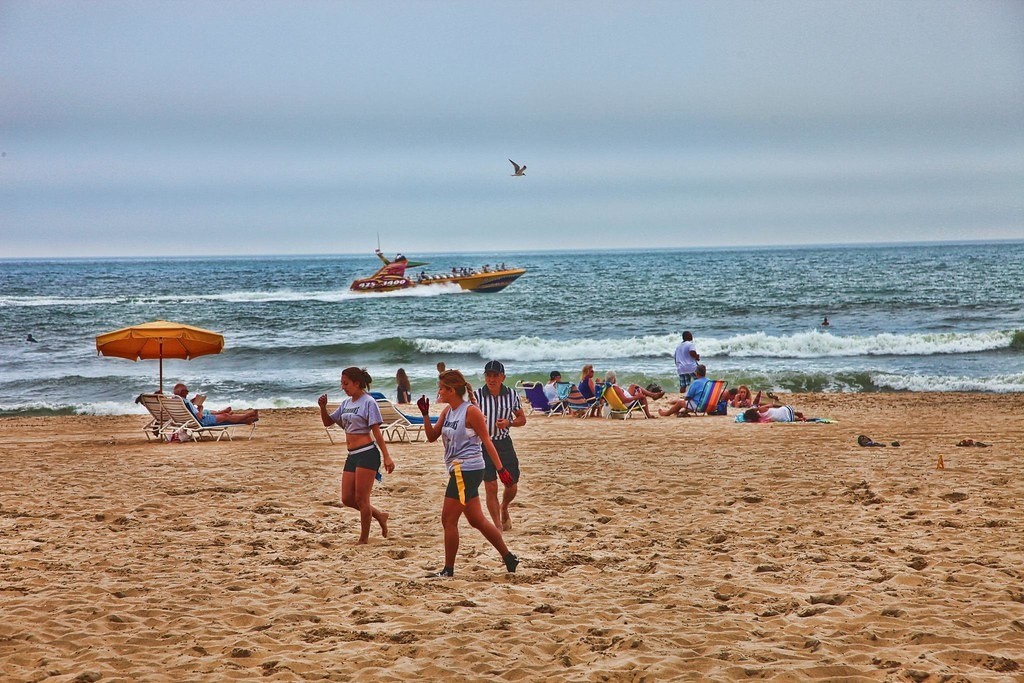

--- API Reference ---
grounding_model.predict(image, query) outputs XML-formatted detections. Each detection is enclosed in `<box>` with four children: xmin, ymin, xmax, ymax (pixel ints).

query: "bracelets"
<box><xmin>423</xmin><ymin>420</ymin><xmax>430</xmax><ymax>423</ymax></box>
<box><xmin>497</xmin><ymin>466</ymin><xmax>505</xmax><ymax>473</ymax></box>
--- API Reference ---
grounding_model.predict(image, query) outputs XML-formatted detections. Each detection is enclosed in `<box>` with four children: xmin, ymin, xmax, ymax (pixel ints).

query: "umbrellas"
<box><xmin>96</xmin><ymin>319</ymin><xmax>225</xmax><ymax>392</ymax></box>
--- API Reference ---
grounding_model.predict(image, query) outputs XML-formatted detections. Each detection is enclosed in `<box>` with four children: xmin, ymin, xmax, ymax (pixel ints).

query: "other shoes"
<box><xmin>425</xmin><ymin>572</ymin><xmax>453</xmax><ymax>578</ymax></box>
<box><xmin>507</xmin><ymin>555</ymin><xmax>519</xmax><ymax>572</ymax></box>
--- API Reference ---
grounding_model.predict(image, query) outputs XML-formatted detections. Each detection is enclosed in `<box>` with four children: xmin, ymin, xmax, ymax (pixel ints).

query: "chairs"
<box><xmin>595</xmin><ymin>381</ymin><xmax>649</xmax><ymax>420</ymax></box>
<box><xmin>680</xmin><ymin>380</ymin><xmax>728</xmax><ymax>417</ymax></box>
<box><xmin>324</xmin><ymin>392</ymin><xmax>441</xmax><ymax>445</ymax></box>
<box><xmin>555</xmin><ymin>381</ymin><xmax>602</xmax><ymax>420</ymax></box>
<box><xmin>515</xmin><ymin>380</ymin><xmax>569</xmax><ymax>417</ymax></box>
<box><xmin>140</xmin><ymin>393</ymin><xmax>259</xmax><ymax>443</ymax></box>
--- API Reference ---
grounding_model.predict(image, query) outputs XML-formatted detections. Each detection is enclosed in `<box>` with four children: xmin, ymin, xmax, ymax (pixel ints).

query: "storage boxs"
<box><xmin>707</xmin><ymin>401</ymin><xmax>727</xmax><ymax>415</ymax></box>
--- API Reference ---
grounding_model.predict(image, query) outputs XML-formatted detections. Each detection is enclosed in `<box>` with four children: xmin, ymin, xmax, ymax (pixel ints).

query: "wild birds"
<box><xmin>508</xmin><ymin>159</ymin><xmax>527</xmax><ymax>177</ymax></box>
<box><xmin>27</xmin><ymin>334</ymin><xmax>38</xmax><ymax>344</ymax></box>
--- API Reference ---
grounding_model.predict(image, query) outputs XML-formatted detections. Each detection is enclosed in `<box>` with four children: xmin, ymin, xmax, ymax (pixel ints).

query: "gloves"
<box><xmin>497</xmin><ymin>467</ymin><xmax>514</xmax><ymax>488</ymax></box>
<box><xmin>417</xmin><ymin>395</ymin><xmax>429</xmax><ymax>416</ymax></box>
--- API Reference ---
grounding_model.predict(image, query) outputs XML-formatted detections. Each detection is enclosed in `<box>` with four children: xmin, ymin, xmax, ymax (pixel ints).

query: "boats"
<box><xmin>348</xmin><ymin>232</ymin><xmax>527</xmax><ymax>293</ymax></box>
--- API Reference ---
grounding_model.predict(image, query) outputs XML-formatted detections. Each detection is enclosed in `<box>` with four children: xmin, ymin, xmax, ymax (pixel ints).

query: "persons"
<box><xmin>396</xmin><ymin>368</ymin><xmax>411</xmax><ymax>404</ymax></box>
<box><xmin>604</xmin><ymin>371</ymin><xmax>665</xmax><ymax>419</ymax></box>
<box><xmin>657</xmin><ymin>365</ymin><xmax>712</xmax><ymax>416</ymax></box>
<box><xmin>543</xmin><ymin>370</ymin><xmax>569</xmax><ymax>412</ymax></box>
<box><xmin>720</xmin><ymin>388</ymin><xmax>738</xmax><ymax>403</ymax></box>
<box><xmin>416</xmin><ymin>370</ymin><xmax>520</xmax><ymax>574</ymax></box>
<box><xmin>153</xmin><ymin>384</ymin><xmax>259</xmax><ymax>425</ymax></box>
<box><xmin>318</xmin><ymin>367</ymin><xmax>395</xmax><ymax>547</ymax></box>
<box><xmin>436</xmin><ymin>361</ymin><xmax>447</xmax><ymax>402</ymax></box>
<box><xmin>580</xmin><ymin>365</ymin><xmax>604</xmax><ymax>418</ymax></box>
<box><xmin>822</xmin><ymin>318</ymin><xmax>829</xmax><ymax>325</ymax></box>
<box><xmin>421</xmin><ymin>262</ymin><xmax>507</xmax><ymax>280</ymax></box>
<box><xmin>731</xmin><ymin>385</ymin><xmax>751</xmax><ymax>408</ymax></box>
<box><xmin>468</xmin><ymin>359</ymin><xmax>526</xmax><ymax>532</ymax></box>
<box><xmin>674</xmin><ymin>331</ymin><xmax>700</xmax><ymax>398</ymax></box>
<box><xmin>743</xmin><ymin>403</ymin><xmax>807</xmax><ymax>422</ymax></box>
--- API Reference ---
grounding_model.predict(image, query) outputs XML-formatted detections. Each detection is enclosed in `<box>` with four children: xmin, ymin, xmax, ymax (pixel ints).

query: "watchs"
<box><xmin>508</xmin><ymin>418</ymin><xmax>514</xmax><ymax>427</ymax></box>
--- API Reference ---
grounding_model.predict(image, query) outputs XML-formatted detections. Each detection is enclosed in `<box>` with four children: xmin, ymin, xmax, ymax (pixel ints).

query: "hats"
<box><xmin>483</xmin><ymin>361</ymin><xmax>505</xmax><ymax>376</ymax></box>
